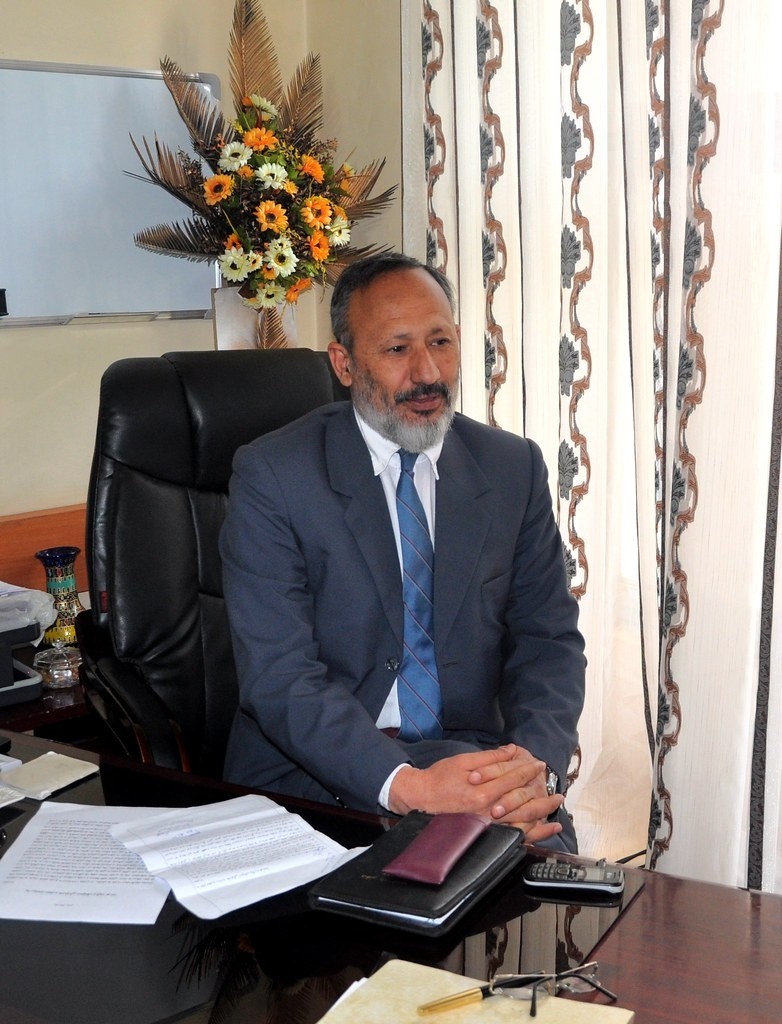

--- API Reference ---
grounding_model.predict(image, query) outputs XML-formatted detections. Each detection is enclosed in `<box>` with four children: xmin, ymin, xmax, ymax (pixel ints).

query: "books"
<box><xmin>306</xmin><ymin>810</ymin><xmax>527</xmax><ymax>938</ymax></box>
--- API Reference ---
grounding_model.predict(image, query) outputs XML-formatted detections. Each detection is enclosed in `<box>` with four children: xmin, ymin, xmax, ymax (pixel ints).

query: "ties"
<box><xmin>395</xmin><ymin>448</ymin><xmax>443</xmax><ymax>743</ymax></box>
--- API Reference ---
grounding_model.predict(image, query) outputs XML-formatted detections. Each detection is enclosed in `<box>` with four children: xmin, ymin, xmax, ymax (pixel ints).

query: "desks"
<box><xmin>0</xmin><ymin>729</ymin><xmax>782</xmax><ymax>1024</ymax></box>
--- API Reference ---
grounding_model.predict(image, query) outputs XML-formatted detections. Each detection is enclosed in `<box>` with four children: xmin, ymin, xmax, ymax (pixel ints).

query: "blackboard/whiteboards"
<box><xmin>1</xmin><ymin>58</ymin><xmax>222</xmax><ymax>329</ymax></box>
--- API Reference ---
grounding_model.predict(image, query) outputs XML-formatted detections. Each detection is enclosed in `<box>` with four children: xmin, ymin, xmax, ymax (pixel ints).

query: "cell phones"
<box><xmin>523</xmin><ymin>862</ymin><xmax>625</xmax><ymax>894</ymax></box>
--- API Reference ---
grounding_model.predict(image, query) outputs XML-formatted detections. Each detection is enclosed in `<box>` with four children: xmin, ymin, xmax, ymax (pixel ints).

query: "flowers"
<box><xmin>121</xmin><ymin>1</ymin><xmax>400</xmax><ymax>349</ymax></box>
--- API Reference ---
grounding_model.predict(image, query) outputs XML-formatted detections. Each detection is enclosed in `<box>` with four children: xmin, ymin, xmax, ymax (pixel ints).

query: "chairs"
<box><xmin>73</xmin><ymin>350</ymin><xmax>350</xmax><ymax>780</ymax></box>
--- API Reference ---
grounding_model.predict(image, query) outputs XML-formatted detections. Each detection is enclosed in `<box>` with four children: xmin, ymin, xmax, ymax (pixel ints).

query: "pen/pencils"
<box><xmin>415</xmin><ymin>971</ymin><xmax>551</xmax><ymax>1018</ymax></box>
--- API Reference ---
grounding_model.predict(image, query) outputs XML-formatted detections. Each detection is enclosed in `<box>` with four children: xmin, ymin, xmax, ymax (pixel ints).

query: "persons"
<box><xmin>217</xmin><ymin>253</ymin><xmax>587</xmax><ymax>856</ymax></box>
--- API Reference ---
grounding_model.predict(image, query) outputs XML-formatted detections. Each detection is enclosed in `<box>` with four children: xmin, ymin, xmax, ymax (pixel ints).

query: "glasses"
<box><xmin>489</xmin><ymin>960</ymin><xmax>618</xmax><ymax>1018</ymax></box>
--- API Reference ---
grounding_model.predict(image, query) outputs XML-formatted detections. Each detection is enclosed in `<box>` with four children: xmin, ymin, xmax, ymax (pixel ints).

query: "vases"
<box><xmin>33</xmin><ymin>544</ymin><xmax>87</xmax><ymax>648</ymax></box>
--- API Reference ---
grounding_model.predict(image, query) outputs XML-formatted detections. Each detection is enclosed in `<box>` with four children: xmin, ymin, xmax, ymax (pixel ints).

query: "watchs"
<box><xmin>547</xmin><ymin>766</ymin><xmax>557</xmax><ymax>795</ymax></box>
